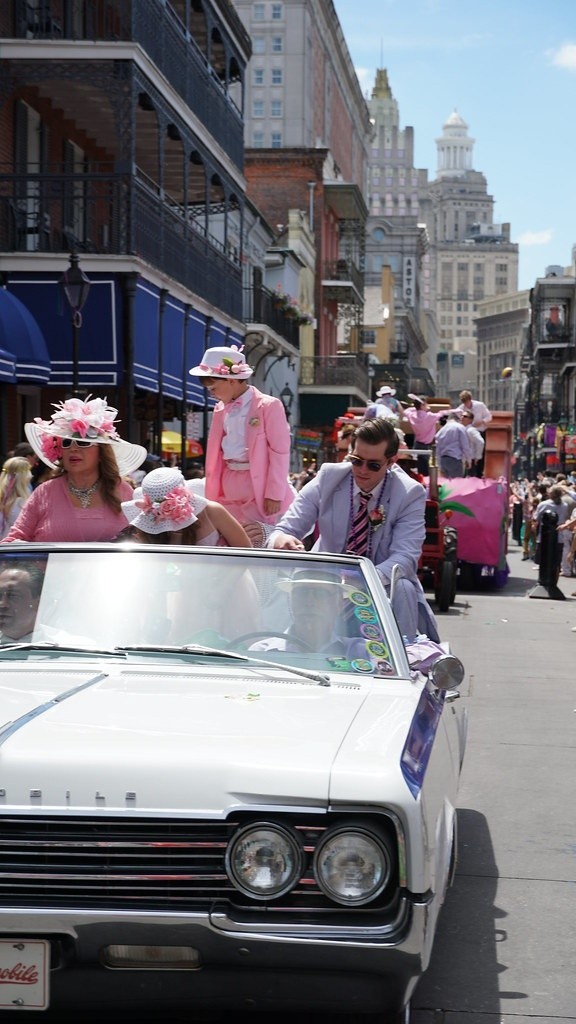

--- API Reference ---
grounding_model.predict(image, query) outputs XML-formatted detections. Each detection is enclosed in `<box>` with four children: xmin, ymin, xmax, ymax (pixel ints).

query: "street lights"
<box><xmin>57</xmin><ymin>244</ymin><xmax>92</xmax><ymax>398</ymax></box>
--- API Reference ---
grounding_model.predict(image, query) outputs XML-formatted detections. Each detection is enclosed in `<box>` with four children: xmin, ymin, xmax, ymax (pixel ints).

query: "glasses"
<box><xmin>292</xmin><ymin>587</ymin><xmax>342</xmax><ymax>601</ymax></box>
<box><xmin>61</xmin><ymin>438</ymin><xmax>99</xmax><ymax>448</ymax></box>
<box><xmin>22</xmin><ymin>450</ymin><xmax>34</xmax><ymax>458</ymax></box>
<box><xmin>461</xmin><ymin>415</ymin><xmax>472</xmax><ymax>419</ymax></box>
<box><xmin>349</xmin><ymin>451</ymin><xmax>391</xmax><ymax>472</ymax></box>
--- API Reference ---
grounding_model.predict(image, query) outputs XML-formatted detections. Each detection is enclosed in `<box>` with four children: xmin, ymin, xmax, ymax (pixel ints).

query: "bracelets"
<box><xmin>252</xmin><ymin>519</ymin><xmax>269</xmax><ymax>549</ymax></box>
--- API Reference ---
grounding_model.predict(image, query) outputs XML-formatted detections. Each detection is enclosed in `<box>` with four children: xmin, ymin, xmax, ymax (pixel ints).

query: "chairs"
<box><xmin>23</xmin><ymin>0</ymin><xmax>64</xmax><ymax>40</ymax></box>
<box><xmin>7</xmin><ymin>195</ymin><xmax>53</xmax><ymax>253</ymax></box>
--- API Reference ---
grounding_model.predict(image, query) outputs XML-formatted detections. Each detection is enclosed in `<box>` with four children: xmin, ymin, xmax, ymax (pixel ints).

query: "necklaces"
<box><xmin>347</xmin><ymin>473</ymin><xmax>388</xmax><ymax>556</ymax></box>
<box><xmin>67</xmin><ymin>477</ymin><xmax>99</xmax><ymax>508</ymax></box>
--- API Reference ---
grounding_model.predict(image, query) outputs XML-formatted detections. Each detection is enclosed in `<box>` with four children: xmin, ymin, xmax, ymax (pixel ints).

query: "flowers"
<box><xmin>368</xmin><ymin>504</ymin><xmax>388</xmax><ymax>532</ymax></box>
<box><xmin>35</xmin><ymin>399</ymin><xmax>121</xmax><ymax>462</ymax></box>
<box><xmin>133</xmin><ymin>484</ymin><xmax>196</xmax><ymax>525</ymax></box>
<box><xmin>271</xmin><ymin>282</ymin><xmax>315</xmax><ymax>326</ymax></box>
<box><xmin>208</xmin><ymin>357</ymin><xmax>245</xmax><ymax>376</ymax></box>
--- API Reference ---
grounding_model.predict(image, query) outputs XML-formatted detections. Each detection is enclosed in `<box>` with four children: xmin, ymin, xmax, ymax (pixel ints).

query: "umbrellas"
<box><xmin>160</xmin><ymin>429</ymin><xmax>205</xmax><ymax>459</ymax></box>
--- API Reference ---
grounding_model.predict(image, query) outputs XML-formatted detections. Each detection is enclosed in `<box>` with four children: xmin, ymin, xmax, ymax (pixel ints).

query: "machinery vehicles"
<box><xmin>327</xmin><ymin>395</ymin><xmax>512</xmax><ymax>614</ymax></box>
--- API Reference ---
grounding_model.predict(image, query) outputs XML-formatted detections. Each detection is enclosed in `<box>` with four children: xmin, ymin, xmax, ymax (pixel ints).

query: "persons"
<box><xmin>242</xmin><ymin>482</ymin><xmax>314</xmax><ymax>547</ymax></box>
<box><xmin>510</xmin><ymin>469</ymin><xmax>576</xmax><ymax>597</ymax></box>
<box><xmin>0</xmin><ymin>397</ymin><xmax>149</xmax><ymax>544</ymax></box>
<box><xmin>246</xmin><ymin>566</ymin><xmax>379</xmax><ymax>661</ymax></box>
<box><xmin>0</xmin><ymin>560</ymin><xmax>76</xmax><ymax>645</ymax></box>
<box><xmin>116</xmin><ymin>468</ymin><xmax>259</xmax><ymax>649</ymax></box>
<box><xmin>267</xmin><ymin>417</ymin><xmax>441</xmax><ymax>648</ymax></box>
<box><xmin>363</xmin><ymin>385</ymin><xmax>493</xmax><ymax>478</ymax></box>
<box><xmin>290</xmin><ymin>462</ymin><xmax>317</xmax><ymax>492</ymax></box>
<box><xmin>105</xmin><ymin>526</ymin><xmax>164</xmax><ymax>599</ymax></box>
<box><xmin>189</xmin><ymin>344</ymin><xmax>298</xmax><ymax>547</ymax></box>
<box><xmin>0</xmin><ymin>442</ymin><xmax>59</xmax><ymax>541</ymax></box>
<box><xmin>122</xmin><ymin>454</ymin><xmax>205</xmax><ymax>490</ymax></box>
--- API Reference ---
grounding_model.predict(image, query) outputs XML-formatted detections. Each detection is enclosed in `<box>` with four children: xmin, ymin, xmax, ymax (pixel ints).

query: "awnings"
<box><xmin>0</xmin><ymin>286</ymin><xmax>52</xmax><ymax>387</ymax></box>
<box><xmin>0</xmin><ymin>350</ymin><xmax>18</xmax><ymax>384</ymax></box>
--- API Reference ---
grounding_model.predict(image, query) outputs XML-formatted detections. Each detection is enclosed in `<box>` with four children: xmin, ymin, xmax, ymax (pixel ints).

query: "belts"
<box><xmin>225</xmin><ymin>460</ymin><xmax>250</xmax><ymax>471</ymax></box>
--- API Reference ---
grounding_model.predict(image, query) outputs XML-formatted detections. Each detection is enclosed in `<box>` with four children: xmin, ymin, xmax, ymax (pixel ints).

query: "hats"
<box><xmin>275</xmin><ymin>567</ymin><xmax>359</xmax><ymax>599</ymax></box>
<box><xmin>14</xmin><ymin>442</ymin><xmax>36</xmax><ymax>457</ymax></box>
<box><xmin>120</xmin><ymin>467</ymin><xmax>211</xmax><ymax>534</ymax></box>
<box><xmin>24</xmin><ymin>393</ymin><xmax>147</xmax><ymax>478</ymax></box>
<box><xmin>190</xmin><ymin>344</ymin><xmax>253</xmax><ymax>379</ymax></box>
<box><xmin>147</xmin><ymin>453</ymin><xmax>160</xmax><ymax>462</ymax></box>
<box><xmin>376</xmin><ymin>385</ymin><xmax>396</xmax><ymax>397</ymax></box>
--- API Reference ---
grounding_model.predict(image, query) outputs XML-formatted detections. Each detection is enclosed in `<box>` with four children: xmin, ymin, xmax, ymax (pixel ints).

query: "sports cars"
<box><xmin>0</xmin><ymin>542</ymin><xmax>466</xmax><ymax>1024</ymax></box>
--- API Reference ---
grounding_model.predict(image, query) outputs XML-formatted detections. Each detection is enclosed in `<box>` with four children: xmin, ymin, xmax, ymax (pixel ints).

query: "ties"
<box><xmin>342</xmin><ymin>492</ymin><xmax>372</xmax><ymax>579</ymax></box>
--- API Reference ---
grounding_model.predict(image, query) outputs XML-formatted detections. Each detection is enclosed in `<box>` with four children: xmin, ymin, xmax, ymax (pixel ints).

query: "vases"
<box><xmin>273</xmin><ymin>302</ymin><xmax>283</xmax><ymax>309</ymax></box>
<box><xmin>283</xmin><ymin>311</ymin><xmax>290</xmax><ymax>320</ymax></box>
